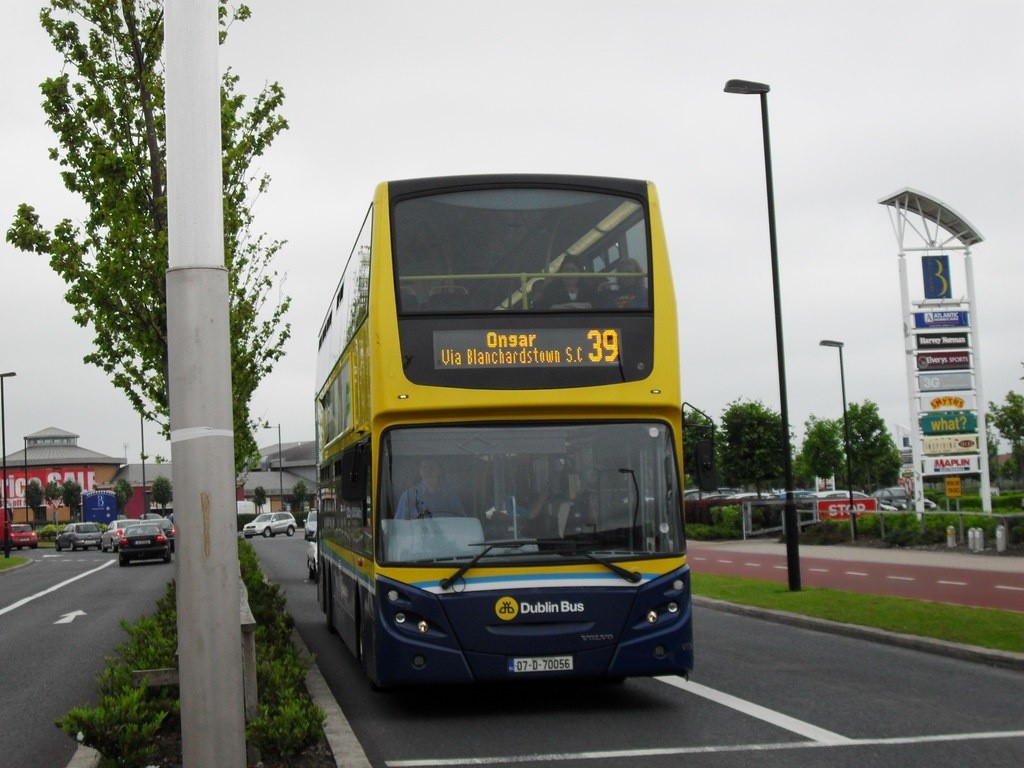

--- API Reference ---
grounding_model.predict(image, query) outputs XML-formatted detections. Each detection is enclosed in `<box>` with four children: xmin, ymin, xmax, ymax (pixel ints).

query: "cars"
<box><xmin>302</xmin><ymin>508</ymin><xmax>318</xmax><ymax>542</ymax></box>
<box><xmin>306</xmin><ymin>527</ymin><xmax>318</xmax><ymax>580</ymax></box>
<box><xmin>100</xmin><ymin>519</ymin><xmax>141</xmax><ymax>553</ymax></box>
<box><xmin>139</xmin><ymin>513</ymin><xmax>176</xmax><ymax>553</ymax></box>
<box><xmin>242</xmin><ymin>512</ymin><xmax>298</xmax><ymax>539</ymax></box>
<box><xmin>685</xmin><ymin>486</ymin><xmax>899</xmax><ymax>512</ymax></box>
<box><xmin>54</xmin><ymin>522</ymin><xmax>103</xmax><ymax>552</ymax></box>
<box><xmin>10</xmin><ymin>524</ymin><xmax>39</xmax><ymax>550</ymax></box>
<box><xmin>872</xmin><ymin>486</ymin><xmax>937</xmax><ymax>513</ymax></box>
<box><xmin>117</xmin><ymin>524</ymin><xmax>172</xmax><ymax>567</ymax></box>
<box><xmin>163</xmin><ymin>513</ymin><xmax>174</xmax><ymax>523</ymax></box>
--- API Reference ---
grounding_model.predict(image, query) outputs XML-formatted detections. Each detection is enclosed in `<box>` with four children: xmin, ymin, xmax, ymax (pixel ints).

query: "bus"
<box><xmin>314</xmin><ymin>174</ymin><xmax>716</xmax><ymax>694</ymax></box>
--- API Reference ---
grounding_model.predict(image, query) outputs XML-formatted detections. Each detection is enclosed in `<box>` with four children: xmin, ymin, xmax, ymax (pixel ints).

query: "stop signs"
<box><xmin>816</xmin><ymin>499</ymin><xmax>875</xmax><ymax>521</ymax></box>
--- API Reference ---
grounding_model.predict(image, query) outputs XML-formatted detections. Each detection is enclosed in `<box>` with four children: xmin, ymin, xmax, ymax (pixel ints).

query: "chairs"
<box><xmin>400</xmin><ymin>275</ymin><xmax>620</xmax><ymax>312</ymax></box>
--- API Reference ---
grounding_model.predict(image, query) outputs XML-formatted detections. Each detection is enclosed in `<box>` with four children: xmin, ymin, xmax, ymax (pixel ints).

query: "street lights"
<box><xmin>723</xmin><ymin>79</ymin><xmax>804</xmax><ymax>595</ymax></box>
<box><xmin>263</xmin><ymin>423</ymin><xmax>285</xmax><ymax>512</ymax></box>
<box><xmin>23</xmin><ymin>436</ymin><xmax>37</xmax><ymax>524</ymax></box>
<box><xmin>819</xmin><ymin>339</ymin><xmax>860</xmax><ymax>544</ymax></box>
<box><xmin>0</xmin><ymin>371</ymin><xmax>17</xmax><ymax>561</ymax></box>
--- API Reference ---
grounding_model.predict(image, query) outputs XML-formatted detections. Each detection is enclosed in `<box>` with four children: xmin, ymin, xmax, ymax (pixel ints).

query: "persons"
<box><xmin>394</xmin><ymin>455</ymin><xmax>468</xmax><ymax>520</ymax></box>
<box><xmin>524</xmin><ymin>475</ymin><xmax>589</xmax><ymax>540</ymax></box>
<box><xmin>532</xmin><ymin>254</ymin><xmax>649</xmax><ymax>308</ymax></box>
